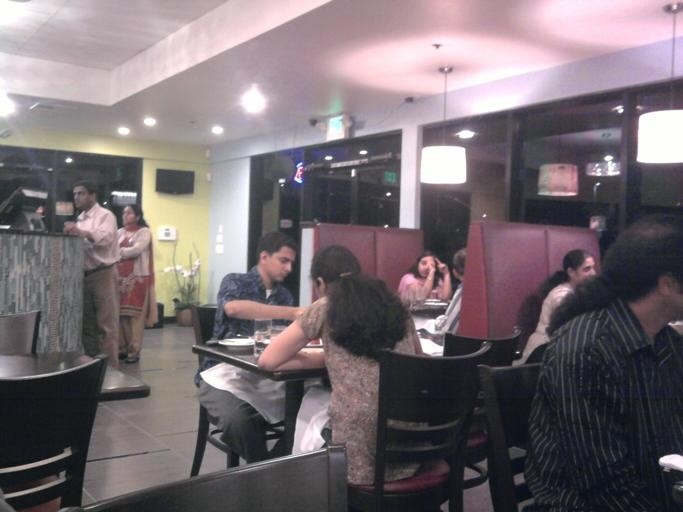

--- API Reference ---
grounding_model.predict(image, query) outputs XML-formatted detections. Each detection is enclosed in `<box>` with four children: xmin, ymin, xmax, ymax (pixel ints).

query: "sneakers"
<box><xmin>119</xmin><ymin>352</ymin><xmax>128</xmax><ymax>360</ymax></box>
<box><xmin>125</xmin><ymin>356</ymin><xmax>139</xmax><ymax>364</ymax></box>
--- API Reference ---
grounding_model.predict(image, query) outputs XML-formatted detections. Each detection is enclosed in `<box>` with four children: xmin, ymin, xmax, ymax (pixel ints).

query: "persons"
<box><xmin>395</xmin><ymin>251</ymin><xmax>453</xmax><ymax>312</ymax></box>
<box><xmin>525</xmin><ymin>213</ymin><xmax>683</xmax><ymax>512</ymax></box>
<box><xmin>63</xmin><ymin>179</ymin><xmax>122</xmax><ymax>374</ymax></box>
<box><xmin>521</xmin><ymin>248</ymin><xmax>597</xmax><ymax>363</ymax></box>
<box><xmin>194</xmin><ymin>230</ymin><xmax>312</xmax><ymax>464</ymax></box>
<box><xmin>430</xmin><ymin>246</ymin><xmax>468</xmax><ymax>335</ymax></box>
<box><xmin>116</xmin><ymin>204</ymin><xmax>152</xmax><ymax>364</ymax></box>
<box><xmin>258</xmin><ymin>245</ymin><xmax>426</xmax><ymax>486</ymax></box>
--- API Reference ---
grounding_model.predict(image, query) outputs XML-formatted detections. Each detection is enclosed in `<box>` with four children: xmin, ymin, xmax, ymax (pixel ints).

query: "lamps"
<box><xmin>537</xmin><ymin>105</ymin><xmax>581</xmax><ymax>198</ymax></box>
<box><xmin>416</xmin><ymin>65</ymin><xmax>469</xmax><ymax>186</ymax></box>
<box><xmin>634</xmin><ymin>1</ymin><xmax>683</xmax><ymax>166</ymax></box>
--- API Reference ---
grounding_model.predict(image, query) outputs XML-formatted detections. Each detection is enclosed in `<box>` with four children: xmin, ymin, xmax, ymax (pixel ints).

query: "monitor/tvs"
<box><xmin>0</xmin><ymin>186</ymin><xmax>48</xmax><ymax>225</ymax></box>
<box><xmin>155</xmin><ymin>168</ymin><xmax>195</xmax><ymax>195</ymax></box>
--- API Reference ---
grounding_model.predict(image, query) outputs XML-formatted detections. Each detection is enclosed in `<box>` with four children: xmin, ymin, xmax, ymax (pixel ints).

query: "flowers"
<box><xmin>160</xmin><ymin>226</ymin><xmax>203</xmax><ymax>311</ymax></box>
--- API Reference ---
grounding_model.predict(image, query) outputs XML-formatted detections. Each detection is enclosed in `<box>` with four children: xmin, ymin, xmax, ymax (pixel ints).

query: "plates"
<box><xmin>218</xmin><ymin>338</ymin><xmax>254</xmax><ymax>347</ymax></box>
<box><xmin>422</xmin><ymin>298</ymin><xmax>448</xmax><ymax>307</ymax></box>
<box><xmin>305</xmin><ymin>343</ymin><xmax>323</xmax><ymax>348</ymax></box>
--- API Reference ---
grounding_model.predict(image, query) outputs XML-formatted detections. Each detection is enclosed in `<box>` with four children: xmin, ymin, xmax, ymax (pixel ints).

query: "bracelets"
<box><xmin>426</xmin><ymin>277</ymin><xmax>434</xmax><ymax>283</ymax></box>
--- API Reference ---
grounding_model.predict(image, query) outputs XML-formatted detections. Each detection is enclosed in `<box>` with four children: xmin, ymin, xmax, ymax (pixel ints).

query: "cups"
<box><xmin>253</xmin><ymin>319</ymin><xmax>271</xmax><ymax>357</ymax></box>
<box><xmin>268</xmin><ymin>325</ymin><xmax>287</xmax><ymax>338</ymax></box>
